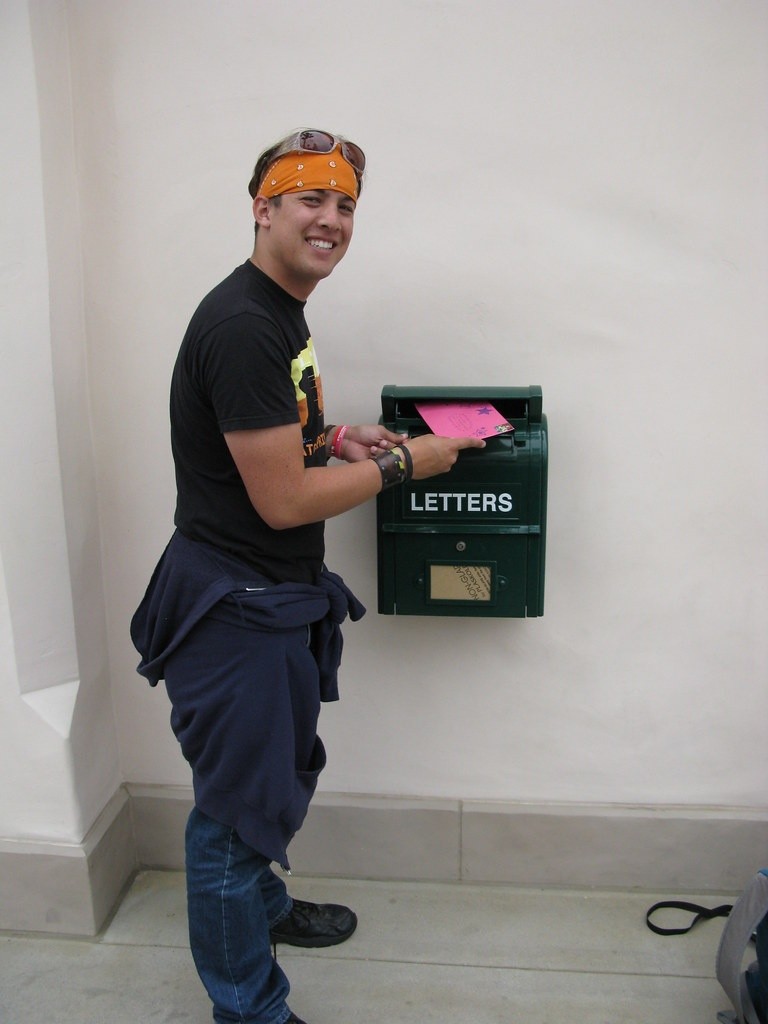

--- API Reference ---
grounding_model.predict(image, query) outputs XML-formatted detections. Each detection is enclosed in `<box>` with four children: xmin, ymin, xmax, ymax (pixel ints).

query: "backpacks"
<box><xmin>716</xmin><ymin>867</ymin><xmax>768</xmax><ymax>1023</ymax></box>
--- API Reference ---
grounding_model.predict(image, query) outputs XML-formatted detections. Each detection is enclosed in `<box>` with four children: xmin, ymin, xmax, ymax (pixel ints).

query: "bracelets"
<box><xmin>374</xmin><ymin>448</ymin><xmax>406</xmax><ymax>492</ymax></box>
<box><xmin>330</xmin><ymin>425</ymin><xmax>350</xmax><ymax>459</ymax></box>
<box><xmin>394</xmin><ymin>444</ymin><xmax>413</xmax><ymax>483</ymax></box>
<box><xmin>324</xmin><ymin>425</ymin><xmax>335</xmax><ymax>439</ymax></box>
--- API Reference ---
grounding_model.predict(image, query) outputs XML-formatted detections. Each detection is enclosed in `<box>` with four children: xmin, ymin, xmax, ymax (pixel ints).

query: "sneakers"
<box><xmin>283</xmin><ymin>1011</ymin><xmax>309</xmax><ymax>1024</ymax></box>
<box><xmin>269</xmin><ymin>899</ymin><xmax>358</xmax><ymax>949</ymax></box>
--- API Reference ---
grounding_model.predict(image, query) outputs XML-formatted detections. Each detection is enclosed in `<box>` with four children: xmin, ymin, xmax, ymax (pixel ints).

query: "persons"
<box><xmin>130</xmin><ymin>127</ymin><xmax>486</xmax><ymax>1024</ymax></box>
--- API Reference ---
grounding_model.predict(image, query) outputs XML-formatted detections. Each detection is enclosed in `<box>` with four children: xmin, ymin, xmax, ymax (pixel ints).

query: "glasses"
<box><xmin>259</xmin><ymin>128</ymin><xmax>365</xmax><ymax>195</ymax></box>
